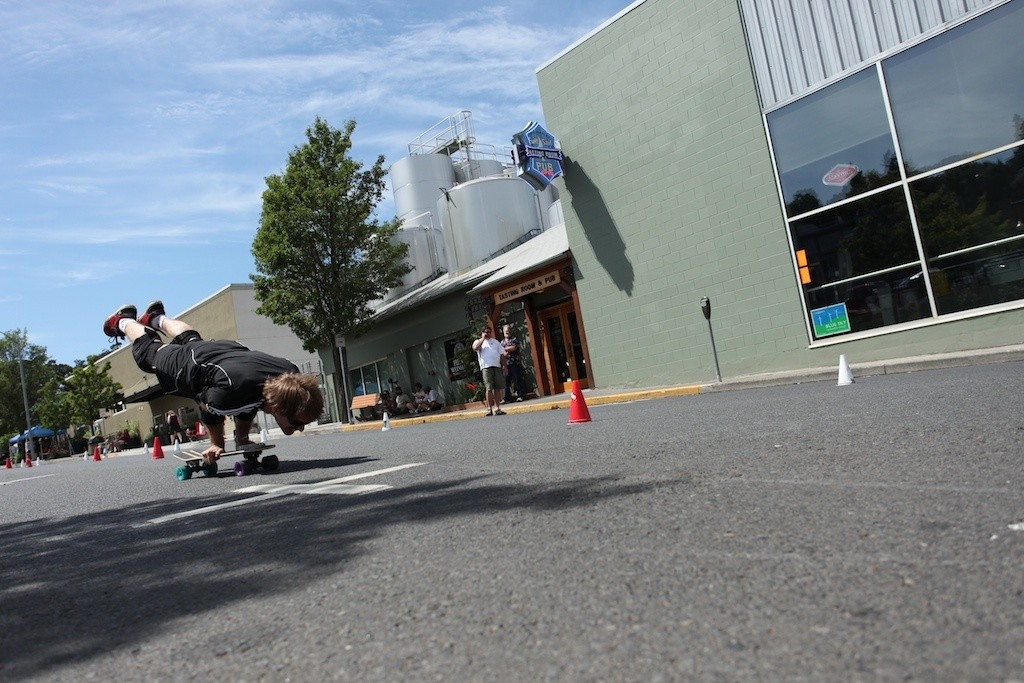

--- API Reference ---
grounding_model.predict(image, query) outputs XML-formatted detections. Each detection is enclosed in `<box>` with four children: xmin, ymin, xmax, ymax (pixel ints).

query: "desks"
<box><xmin>878</xmin><ymin>287</ymin><xmax>921</xmax><ymax>325</ymax></box>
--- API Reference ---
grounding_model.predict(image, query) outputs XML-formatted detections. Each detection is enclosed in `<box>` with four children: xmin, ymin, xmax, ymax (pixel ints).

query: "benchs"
<box><xmin>349</xmin><ymin>393</ymin><xmax>380</xmax><ymax>424</ymax></box>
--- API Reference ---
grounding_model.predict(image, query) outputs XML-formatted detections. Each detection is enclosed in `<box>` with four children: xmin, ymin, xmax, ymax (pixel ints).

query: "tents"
<box><xmin>9</xmin><ymin>424</ymin><xmax>74</xmax><ymax>467</ymax></box>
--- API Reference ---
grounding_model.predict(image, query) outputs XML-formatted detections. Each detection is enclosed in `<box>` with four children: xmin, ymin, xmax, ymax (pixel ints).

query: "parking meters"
<box><xmin>700</xmin><ymin>297</ymin><xmax>723</xmax><ymax>382</ymax></box>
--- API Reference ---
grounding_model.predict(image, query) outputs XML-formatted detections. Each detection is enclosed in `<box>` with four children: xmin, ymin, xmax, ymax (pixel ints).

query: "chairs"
<box><xmin>120</xmin><ymin>435</ymin><xmax>130</xmax><ymax>452</ymax></box>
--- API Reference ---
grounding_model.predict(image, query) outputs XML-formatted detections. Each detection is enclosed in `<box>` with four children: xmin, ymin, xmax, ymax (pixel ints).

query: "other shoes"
<box><xmin>486</xmin><ymin>409</ymin><xmax>493</xmax><ymax>416</ymax></box>
<box><xmin>517</xmin><ymin>397</ymin><xmax>522</xmax><ymax>402</ymax></box>
<box><xmin>499</xmin><ymin>400</ymin><xmax>510</xmax><ymax>404</ymax></box>
<box><xmin>495</xmin><ymin>409</ymin><xmax>507</xmax><ymax>415</ymax></box>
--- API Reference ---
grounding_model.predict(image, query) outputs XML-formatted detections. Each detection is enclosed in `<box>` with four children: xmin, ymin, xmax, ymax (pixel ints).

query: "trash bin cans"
<box><xmin>87</xmin><ymin>436</ymin><xmax>105</xmax><ymax>456</ymax></box>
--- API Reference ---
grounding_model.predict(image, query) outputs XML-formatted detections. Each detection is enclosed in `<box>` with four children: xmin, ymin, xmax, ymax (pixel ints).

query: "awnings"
<box><xmin>467</xmin><ymin>225</ymin><xmax>570</xmax><ymax>295</ymax></box>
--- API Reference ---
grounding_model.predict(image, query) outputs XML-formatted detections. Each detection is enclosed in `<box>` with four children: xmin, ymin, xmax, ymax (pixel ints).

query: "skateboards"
<box><xmin>175</xmin><ymin>444</ymin><xmax>280</xmax><ymax>481</ymax></box>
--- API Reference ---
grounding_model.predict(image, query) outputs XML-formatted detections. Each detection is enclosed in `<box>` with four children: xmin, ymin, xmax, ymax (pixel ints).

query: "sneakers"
<box><xmin>103</xmin><ymin>304</ymin><xmax>137</xmax><ymax>342</ymax></box>
<box><xmin>136</xmin><ymin>300</ymin><xmax>165</xmax><ymax>330</ymax></box>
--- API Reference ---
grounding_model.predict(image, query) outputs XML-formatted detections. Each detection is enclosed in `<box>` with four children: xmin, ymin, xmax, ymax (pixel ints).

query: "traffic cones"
<box><xmin>93</xmin><ymin>447</ymin><xmax>101</xmax><ymax>462</ymax></box>
<box><xmin>174</xmin><ymin>439</ymin><xmax>181</xmax><ymax>451</ymax></box>
<box><xmin>6</xmin><ymin>458</ymin><xmax>12</xmax><ymax>468</ymax></box>
<box><xmin>84</xmin><ymin>452</ymin><xmax>89</xmax><ymax>462</ymax></box>
<box><xmin>382</xmin><ymin>412</ymin><xmax>393</xmax><ymax>432</ymax></box>
<box><xmin>835</xmin><ymin>353</ymin><xmax>856</xmax><ymax>387</ymax></box>
<box><xmin>259</xmin><ymin>428</ymin><xmax>269</xmax><ymax>443</ymax></box>
<box><xmin>153</xmin><ymin>437</ymin><xmax>164</xmax><ymax>459</ymax></box>
<box><xmin>142</xmin><ymin>443</ymin><xmax>151</xmax><ymax>455</ymax></box>
<box><xmin>36</xmin><ymin>457</ymin><xmax>41</xmax><ymax>466</ymax></box>
<box><xmin>569</xmin><ymin>380</ymin><xmax>592</xmax><ymax>424</ymax></box>
<box><xmin>26</xmin><ymin>455</ymin><xmax>33</xmax><ymax>468</ymax></box>
<box><xmin>20</xmin><ymin>460</ymin><xmax>25</xmax><ymax>468</ymax></box>
<box><xmin>104</xmin><ymin>449</ymin><xmax>109</xmax><ymax>461</ymax></box>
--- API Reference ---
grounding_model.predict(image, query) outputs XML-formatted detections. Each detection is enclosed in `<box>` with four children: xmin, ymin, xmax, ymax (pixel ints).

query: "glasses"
<box><xmin>288</xmin><ymin>415</ymin><xmax>306</xmax><ymax>427</ymax></box>
<box><xmin>485</xmin><ymin>331</ymin><xmax>491</xmax><ymax>333</ymax></box>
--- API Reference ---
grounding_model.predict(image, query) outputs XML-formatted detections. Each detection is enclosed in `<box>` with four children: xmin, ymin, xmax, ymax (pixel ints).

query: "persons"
<box><xmin>472</xmin><ymin>326</ymin><xmax>508</xmax><ymax>415</ymax></box>
<box><xmin>823</xmin><ymin>238</ymin><xmax>872</xmax><ymax>307</ymax></box>
<box><xmin>167</xmin><ymin>409</ymin><xmax>182</xmax><ymax>444</ymax></box>
<box><xmin>406</xmin><ymin>382</ymin><xmax>426</xmax><ymax>413</ymax></box>
<box><xmin>103</xmin><ymin>299</ymin><xmax>324</xmax><ymax>464</ymax></box>
<box><xmin>418</xmin><ymin>385</ymin><xmax>444</xmax><ymax>412</ymax></box>
<box><xmin>106</xmin><ymin>429</ymin><xmax>130</xmax><ymax>453</ymax></box>
<box><xmin>355</xmin><ymin>390</ymin><xmax>396</xmax><ymax>422</ymax></box>
<box><xmin>384</xmin><ymin>386</ymin><xmax>411</xmax><ymax>416</ymax></box>
<box><xmin>9</xmin><ymin>439</ymin><xmax>56</xmax><ymax>463</ymax></box>
<box><xmin>501</xmin><ymin>324</ymin><xmax>525</xmax><ymax>404</ymax></box>
